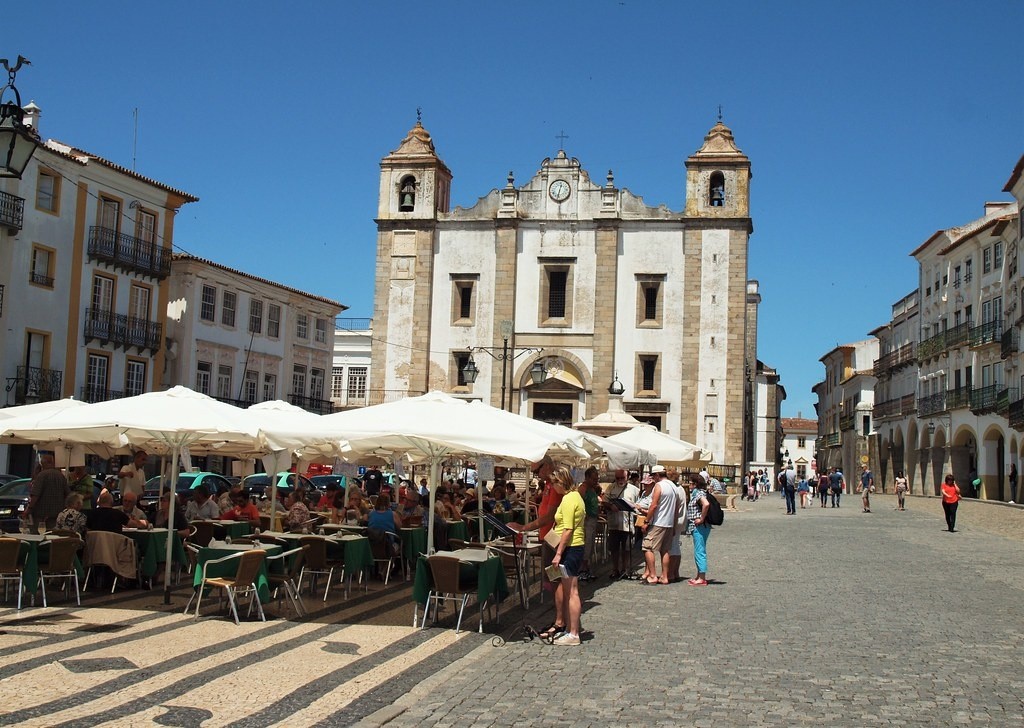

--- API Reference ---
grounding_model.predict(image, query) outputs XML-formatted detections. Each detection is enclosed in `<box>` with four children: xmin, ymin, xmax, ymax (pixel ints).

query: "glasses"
<box><xmin>615</xmin><ymin>476</ymin><xmax>627</xmax><ymax>480</ymax></box>
<box><xmin>534</xmin><ymin>460</ymin><xmax>546</xmax><ymax>476</ymax></box>
<box><xmin>403</xmin><ymin>496</ymin><xmax>418</xmax><ymax>504</ymax></box>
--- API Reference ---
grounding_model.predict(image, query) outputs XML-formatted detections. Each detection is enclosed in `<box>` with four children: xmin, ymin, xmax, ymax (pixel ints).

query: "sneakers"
<box><xmin>553</xmin><ymin>634</ymin><xmax>581</xmax><ymax>645</ymax></box>
<box><xmin>547</xmin><ymin>630</ymin><xmax>568</xmax><ymax>643</ymax></box>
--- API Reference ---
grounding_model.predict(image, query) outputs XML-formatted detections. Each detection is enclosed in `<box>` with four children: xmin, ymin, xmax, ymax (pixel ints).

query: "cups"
<box><xmin>337</xmin><ymin>531</ymin><xmax>342</xmax><ymax>538</ymax></box>
<box><xmin>254</xmin><ymin>540</ymin><xmax>260</xmax><ymax>548</ymax></box>
<box><xmin>302</xmin><ymin>528</ymin><xmax>308</xmax><ymax>535</ymax></box>
<box><xmin>428</xmin><ymin>546</ymin><xmax>435</xmax><ymax>557</ymax></box>
<box><xmin>319</xmin><ymin>528</ymin><xmax>325</xmax><ymax>536</ymax></box>
<box><xmin>38</xmin><ymin>522</ymin><xmax>46</xmax><ymax>536</ymax></box>
<box><xmin>148</xmin><ymin>523</ymin><xmax>153</xmax><ymax>532</ymax></box>
<box><xmin>19</xmin><ymin>520</ymin><xmax>28</xmax><ymax>534</ymax></box>
<box><xmin>255</xmin><ymin>528</ymin><xmax>260</xmax><ymax>534</ymax></box>
<box><xmin>226</xmin><ymin>536</ymin><xmax>231</xmax><ymax>545</ymax></box>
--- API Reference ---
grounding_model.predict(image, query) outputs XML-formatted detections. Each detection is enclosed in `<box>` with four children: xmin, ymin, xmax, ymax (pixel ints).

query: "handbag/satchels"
<box><xmin>748</xmin><ymin>486</ymin><xmax>754</xmax><ymax>496</ymax></box>
<box><xmin>954</xmin><ymin>484</ymin><xmax>963</xmax><ymax>500</ymax></box>
<box><xmin>869</xmin><ymin>485</ymin><xmax>875</xmax><ymax>494</ymax></box>
<box><xmin>818</xmin><ymin>484</ymin><xmax>824</xmax><ymax>491</ymax></box>
<box><xmin>780</xmin><ymin>469</ymin><xmax>787</xmax><ymax>486</ymax></box>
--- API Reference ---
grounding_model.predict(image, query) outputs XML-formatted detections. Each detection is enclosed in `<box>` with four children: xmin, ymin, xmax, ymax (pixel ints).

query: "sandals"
<box><xmin>541</xmin><ymin>625</ymin><xmax>566</xmax><ymax>639</ymax></box>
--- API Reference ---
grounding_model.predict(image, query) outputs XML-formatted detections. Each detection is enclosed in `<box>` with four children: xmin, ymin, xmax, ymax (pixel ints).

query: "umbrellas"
<box><xmin>245</xmin><ymin>390</ymin><xmax>716</xmax><ymax>552</ymax></box>
<box><xmin>0</xmin><ymin>385</ymin><xmax>271</xmax><ymax>603</ymax></box>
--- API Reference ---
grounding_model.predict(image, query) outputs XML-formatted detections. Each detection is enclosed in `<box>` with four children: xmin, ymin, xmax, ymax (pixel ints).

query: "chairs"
<box><xmin>0</xmin><ymin>494</ymin><xmax>593</xmax><ymax>635</ymax></box>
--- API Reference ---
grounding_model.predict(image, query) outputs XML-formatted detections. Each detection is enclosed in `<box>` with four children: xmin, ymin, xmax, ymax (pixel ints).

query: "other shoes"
<box><xmin>610</xmin><ymin>569</ymin><xmax>629</xmax><ymax>579</ymax></box>
<box><xmin>862</xmin><ymin>509</ymin><xmax>871</xmax><ymax>513</ymax></box>
<box><xmin>577</xmin><ymin>571</ymin><xmax>597</xmax><ymax>581</ymax></box>
<box><xmin>688</xmin><ymin>576</ymin><xmax>708</xmax><ymax>585</ymax></box>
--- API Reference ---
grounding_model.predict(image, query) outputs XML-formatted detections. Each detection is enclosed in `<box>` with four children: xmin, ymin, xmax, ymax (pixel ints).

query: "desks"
<box><xmin>438</xmin><ymin>517</ymin><xmax>468</xmax><ymax>542</ymax></box>
<box><xmin>411</xmin><ymin>549</ymin><xmax>501</xmax><ymax>633</ymax></box>
<box><xmin>308</xmin><ymin>509</ymin><xmax>346</xmax><ymax>523</ymax></box>
<box><xmin>314</xmin><ymin>523</ymin><xmax>426</xmax><ymax>584</ymax></box>
<box><xmin>181</xmin><ymin>542</ymin><xmax>281</xmax><ymax>625</ymax></box>
<box><xmin>249</xmin><ymin>532</ymin><xmax>368</xmax><ymax>601</ymax></box>
<box><xmin>255</xmin><ymin>510</ymin><xmax>290</xmax><ymax>531</ymax></box>
<box><xmin>517</xmin><ymin>531</ymin><xmax>540</xmax><ymax>582</ymax></box>
<box><xmin>1</xmin><ymin>533</ymin><xmax>84</xmax><ymax>605</ymax></box>
<box><xmin>188</xmin><ymin>519</ymin><xmax>252</xmax><ymax>542</ymax></box>
<box><xmin>121</xmin><ymin>527</ymin><xmax>187</xmax><ymax>590</ymax></box>
<box><xmin>468</xmin><ymin>516</ymin><xmax>495</xmax><ymax>540</ymax></box>
<box><xmin>510</xmin><ymin>506</ymin><xmax>529</xmax><ymax>524</ymax></box>
<box><xmin>474</xmin><ymin>540</ymin><xmax>543</xmax><ymax>609</ymax></box>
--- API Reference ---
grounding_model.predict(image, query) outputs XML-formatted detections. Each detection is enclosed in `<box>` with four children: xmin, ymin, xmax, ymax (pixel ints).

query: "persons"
<box><xmin>1009</xmin><ymin>463</ymin><xmax>1017</xmax><ymax>501</ymax></box>
<box><xmin>579</xmin><ymin>465</ymin><xmax>686</xmax><ymax>585</ymax></box>
<box><xmin>688</xmin><ymin>473</ymin><xmax>711</xmax><ymax>585</ymax></box>
<box><xmin>741</xmin><ymin>468</ymin><xmax>771</xmax><ymax>502</ymax></box>
<box><xmin>23</xmin><ymin>449</ymin><xmax>545</xmax><ymax>585</ymax></box>
<box><xmin>969</xmin><ymin>469</ymin><xmax>976</xmax><ymax>497</ymax></box>
<box><xmin>856</xmin><ymin>464</ymin><xmax>873</xmax><ymax>513</ymax></box>
<box><xmin>778</xmin><ymin>465</ymin><xmax>843</xmax><ymax>515</ymax></box>
<box><xmin>941</xmin><ymin>475</ymin><xmax>960</xmax><ymax>531</ymax></box>
<box><xmin>894</xmin><ymin>471</ymin><xmax>909</xmax><ymax>511</ymax></box>
<box><xmin>700</xmin><ymin>468</ymin><xmax>728</xmax><ymax>494</ymax></box>
<box><xmin>521</xmin><ymin>456</ymin><xmax>585</xmax><ymax>645</ymax></box>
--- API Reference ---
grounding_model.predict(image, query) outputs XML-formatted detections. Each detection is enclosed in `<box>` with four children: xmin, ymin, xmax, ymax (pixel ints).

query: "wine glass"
<box><xmin>488</xmin><ymin>530</ymin><xmax>493</xmax><ymax>547</ymax></box>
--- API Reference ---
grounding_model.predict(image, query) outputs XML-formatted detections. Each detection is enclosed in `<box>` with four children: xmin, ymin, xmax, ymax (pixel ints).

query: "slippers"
<box><xmin>640</xmin><ymin>576</ymin><xmax>657</xmax><ymax>586</ymax></box>
<box><xmin>658</xmin><ymin>576</ymin><xmax>668</xmax><ymax>584</ymax></box>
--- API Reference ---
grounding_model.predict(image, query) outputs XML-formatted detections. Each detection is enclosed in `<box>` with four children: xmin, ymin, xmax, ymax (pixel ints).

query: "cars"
<box><xmin>0</xmin><ymin>471</ymin><xmax>405</xmax><ymax>527</ymax></box>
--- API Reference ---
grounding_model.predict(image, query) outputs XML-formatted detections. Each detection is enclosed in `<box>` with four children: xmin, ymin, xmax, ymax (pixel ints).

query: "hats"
<box><xmin>529</xmin><ymin>484</ymin><xmax>537</xmax><ymax>489</ymax></box>
<box><xmin>466</xmin><ymin>488</ymin><xmax>474</xmax><ymax>497</ymax></box>
<box><xmin>648</xmin><ymin>465</ymin><xmax>667</xmax><ymax>475</ymax></box>
<box><xmin>640</xmin><ymin>475</ymin><xmax>654</xmax><ymax>485</ymax></box>
<box><xmin>629</xmin><ymin>472</ymin><xmax>638</xmax><ymax>479</ymax></box>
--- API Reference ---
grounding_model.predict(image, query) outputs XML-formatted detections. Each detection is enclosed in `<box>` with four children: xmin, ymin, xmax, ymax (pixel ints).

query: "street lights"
<box><xmin>462</xmin><ymin>320</ymin><xmax>548</xmax><ymax>410</ymax></box>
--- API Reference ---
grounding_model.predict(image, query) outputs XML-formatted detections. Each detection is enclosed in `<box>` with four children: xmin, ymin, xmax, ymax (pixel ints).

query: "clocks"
<box><xmin>549</xmin><ymin>178</ymin><xmax>570</xmax><ymax>201</ymax></box>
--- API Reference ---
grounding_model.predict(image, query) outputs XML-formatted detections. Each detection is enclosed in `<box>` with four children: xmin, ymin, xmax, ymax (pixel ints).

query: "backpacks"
<box><xmin>695</xmin><ymin>490</ymin><xmax>724</xmax><ymax>526</ymax></box>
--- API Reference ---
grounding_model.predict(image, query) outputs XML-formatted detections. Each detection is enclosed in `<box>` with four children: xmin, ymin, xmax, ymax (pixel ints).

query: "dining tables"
<box><xmin>494</xmin><ymin>510</ymin><xmax>512</xmax><ymax>527</ymax></box>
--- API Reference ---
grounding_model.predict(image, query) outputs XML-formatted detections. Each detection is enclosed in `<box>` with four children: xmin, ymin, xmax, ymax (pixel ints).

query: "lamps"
<box><xmin>780</xmin><ymin>450</ymin><xmax>789</xmax><ymax>457</ymax></box>
<box><xmin>928</xmin><ymin>413</ymin><xmax>936</xmax><ymax>434</ymax></box>
<box><xmin>788</xmin><ymin>459</ymin><xmax>792</xmax><ymax>465</ymax></box>
<box><xmin>0</xmin><ymin>53</ymin><xmax>41</xmax><ymax>181</ymax></box>
<box><xmin>818</xmin><ymin>433</ymin><xmax>823</xmax><ymax>440</ymax></box>
